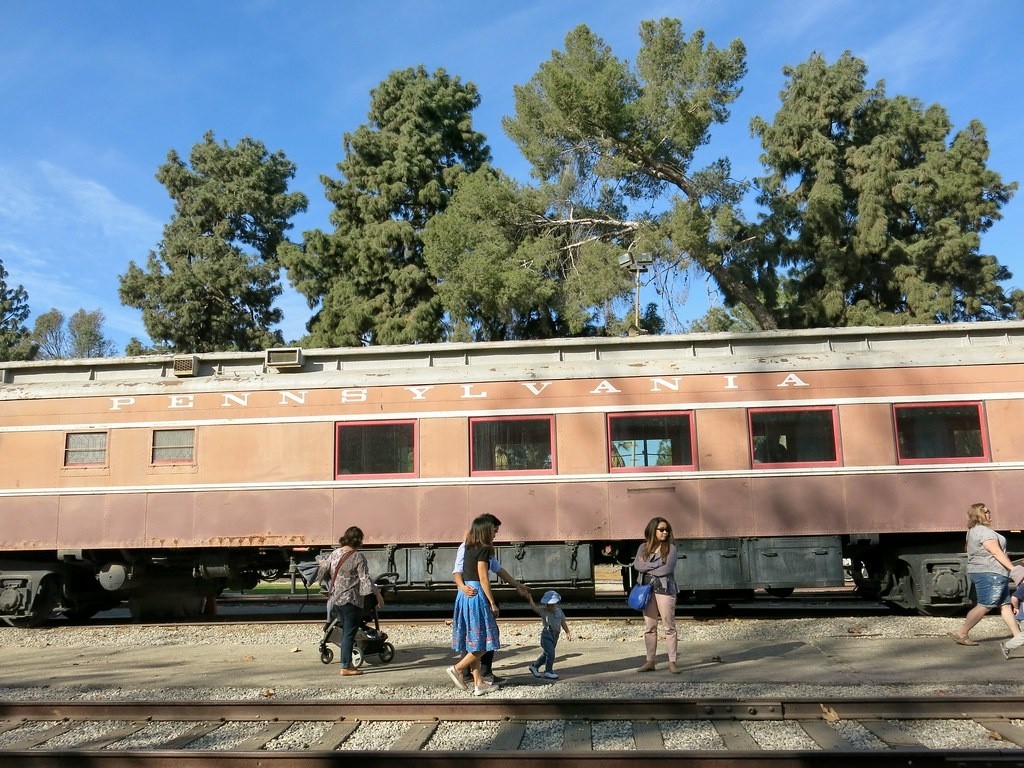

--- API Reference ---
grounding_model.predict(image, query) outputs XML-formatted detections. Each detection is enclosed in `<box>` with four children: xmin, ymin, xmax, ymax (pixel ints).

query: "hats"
<box><xmin>540</xmin><ymin>590</ymin><xmax>562</xmax><ymax>605</ymax></box>
<box><xmin>1010</xmin><ymin>565</ymin><xmax>1024</xmax><ymax>586</ymax></box>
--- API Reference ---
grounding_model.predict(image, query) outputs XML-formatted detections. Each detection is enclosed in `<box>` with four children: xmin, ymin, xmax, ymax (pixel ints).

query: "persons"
<box><xmin>999</xmin><ymin>565</ymin><xmax>1023</xmax><ymax>659</ymax></box>
<box><xmin>522</xmin><ymin>591</ymin><xmax>573</xmax><ymax>678</ymax></box>
<box><xmin>445</xmin><ymin>513</ymin><xmax>530</xmax><ymax>696</ymax></box>
<box><xmin>316</xmin><ymin>526</ymin><xmax>384</xmax><ymax>676</ymax></box>
<box><xmin>633</xmin><ymin>517</ymin><xmax>682</xmax><ymax>674</ymax></box>
<box><xmin>952</xmin><ymin>503</ymin><xmax>1024</xmax><ymax>660</ymax></box>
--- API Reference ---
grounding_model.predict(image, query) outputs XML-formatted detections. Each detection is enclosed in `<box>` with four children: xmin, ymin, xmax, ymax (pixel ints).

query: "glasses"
<box><xmin>985</xmin><ymin>510</ymin><xmax>991</xmax><ymax>515</ymax></box>
<box><xmin>656</xmin><ymin>528</ymin><xmax>669</xmax><ymax>532</ymax></box>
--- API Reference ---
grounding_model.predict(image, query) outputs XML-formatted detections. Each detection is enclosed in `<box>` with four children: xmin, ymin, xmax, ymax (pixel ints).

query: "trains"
<box><xmin>0</xmin><ymin>320</ymin><xmax>1022</xmax><ymax>629</ymax></box>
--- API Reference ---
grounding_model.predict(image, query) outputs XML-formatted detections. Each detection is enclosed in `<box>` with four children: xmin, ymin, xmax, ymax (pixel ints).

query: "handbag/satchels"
<box><xmin>627</xmin><ymin>582</ymin><xmax>655</xmax><ymax>610</ymax></box>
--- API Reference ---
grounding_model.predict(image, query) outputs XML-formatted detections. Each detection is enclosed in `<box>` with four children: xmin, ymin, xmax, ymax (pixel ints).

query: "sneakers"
<box><xmin>544</xmin><ymin>672</ymin><xmax>559</xmax><ymax>679</ymax></box>
<box><xmin>479</xmin><ymin>673</ymin><xmax>507</xmax><ymax>686</ymax></box>
<box><xmin>474</xmin><ymin>682</ymin><xmax>500</xmax><ymax>696</ymax></box>
<box><xmin>447</xmin><ymin>666</ymin><xmax>468</xmax><ymax>691</ymax></box>
<box><xmin>529</xmin><ymin>665</ymin><xmax>541</xmax><ymax>678</ymax></box>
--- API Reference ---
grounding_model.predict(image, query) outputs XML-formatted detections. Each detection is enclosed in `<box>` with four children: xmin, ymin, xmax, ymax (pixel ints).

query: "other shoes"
<box><xmin>636</xmin><ymin>664</ymin><xmax>656</xmax><ymax>672</ymax></box>
<box><xmin>669</xmin><ymin>665</ymin><xmax>682</xmax><ymax>674</ymax></box>
<box><xmin>948</xmin><ymin>631</ymin><xmax>979</xmax><ymax>647</ymax></box>
<box><xmin>341</xmin><ymin>668</ymin><xmax>363</xmax><ymax>676</ymax></box>
<box><xmin>1001</xmin><ymin>641</ymin><xmax>1012</xmax><ymax>659</ymax></box>
<box><xmin>461</xmin><ymin>673</ymin><xmax>475</xmax><ymax>684</ymax></box>
